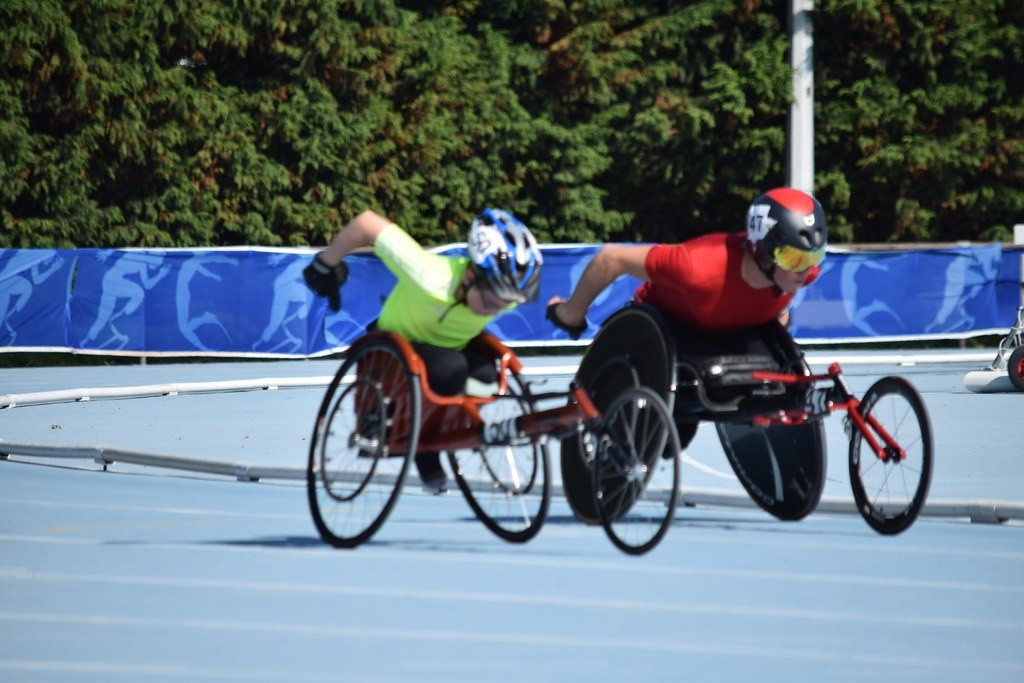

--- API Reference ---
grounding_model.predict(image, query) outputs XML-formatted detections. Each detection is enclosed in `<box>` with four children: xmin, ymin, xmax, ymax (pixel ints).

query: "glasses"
<box><xmin>774</xmin><ymin>246</ymin><xmax>827</xmax><ymax>271</ymax></box>
<box><xmin>475</xmin><ymin>277</ymin><xmax>510</xmax><ymax>313</ymax></box>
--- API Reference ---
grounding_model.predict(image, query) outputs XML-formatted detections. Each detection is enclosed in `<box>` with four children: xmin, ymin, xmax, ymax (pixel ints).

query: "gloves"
<box><xmin>305</xmin><ymin>251</ymin><xmax>349</xmax><ymax>312</ymax></box>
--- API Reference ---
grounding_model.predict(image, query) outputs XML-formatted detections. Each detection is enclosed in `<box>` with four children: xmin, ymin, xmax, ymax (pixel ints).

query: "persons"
<box><xmin>303</xmin><ymin>208</ymin><xmax>543</xmax><ymax>396</ymax></box>
<box><xmin>546</xmin><ymin>188</ymin><xmax>829</xmax><ymax>359</ymax></box>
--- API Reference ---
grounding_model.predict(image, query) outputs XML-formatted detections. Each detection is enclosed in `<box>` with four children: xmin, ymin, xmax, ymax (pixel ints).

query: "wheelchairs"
<box><xmin>308</xmin><ymin>330</ymin><xmax>552</xmax><ymax>549</ymax></box>
<box><xmin>559</xmin><ymin>304</ymin><xmax>935</xmax><ymax>555</ymax></box>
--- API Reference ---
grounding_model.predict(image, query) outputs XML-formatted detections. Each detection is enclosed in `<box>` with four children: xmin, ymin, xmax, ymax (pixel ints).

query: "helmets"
<box><xmin>750</xmin><ymin>187</ymin><xmax>827</xmax><ymax>281</ymax></box>
<box><xmin>467</xmin><ymin>206</ymin><xmax>543</xmax><ymax>303</ymax></box>
